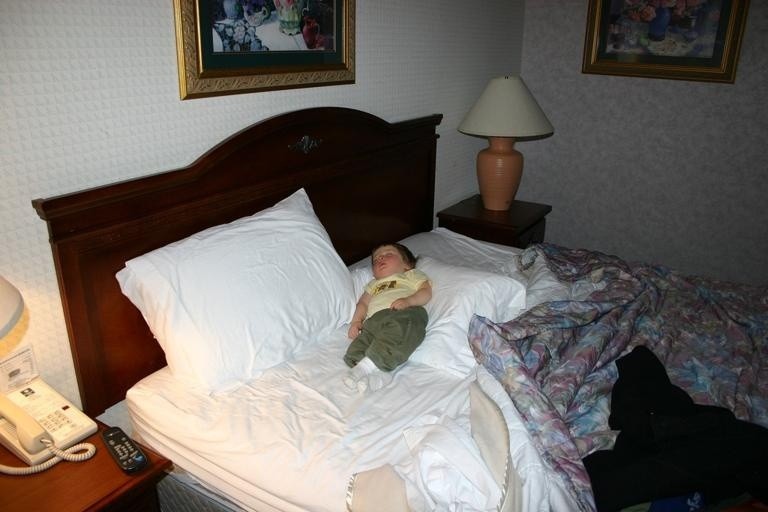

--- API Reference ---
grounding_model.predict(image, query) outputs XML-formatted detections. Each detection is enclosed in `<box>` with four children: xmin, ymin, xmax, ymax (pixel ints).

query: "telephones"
<box><xmin>0</xmin><ymin>376</ymin><xmax>98</xmax><ymax>466</ymax></box>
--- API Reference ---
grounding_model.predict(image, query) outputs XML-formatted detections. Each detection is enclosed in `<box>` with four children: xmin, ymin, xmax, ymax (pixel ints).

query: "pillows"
<box><xmin>114</xmin><ymin>188</ymin><xmax>359</xmax><ymax>395</ymax></box>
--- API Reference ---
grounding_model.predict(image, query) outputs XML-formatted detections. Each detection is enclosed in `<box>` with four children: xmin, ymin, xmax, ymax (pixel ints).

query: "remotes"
<box><xmin>100</xmin><ymin>426</ymin><xmax>148</xmax><ymax>473</ymax></box>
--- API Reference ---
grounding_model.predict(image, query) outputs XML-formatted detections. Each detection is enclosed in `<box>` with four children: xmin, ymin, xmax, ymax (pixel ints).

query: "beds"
<box><xmin>29</xmin><ymin>106</ymin><xmax>767</xmax><ymax>512</ymax></box>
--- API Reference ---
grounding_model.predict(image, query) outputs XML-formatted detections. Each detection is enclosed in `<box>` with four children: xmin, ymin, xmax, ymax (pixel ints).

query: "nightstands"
<box><xmin>0</xmin><ymin>413</ymin><xmax>176</xmax><ymax>512</ymax></box>
<box><xmin>436</xmin><ymin>194</ymin><xmax>556</xmax><ymax>252</ymax></box>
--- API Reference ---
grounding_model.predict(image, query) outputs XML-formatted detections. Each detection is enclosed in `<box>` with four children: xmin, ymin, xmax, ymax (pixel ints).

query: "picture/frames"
<box><xmin>580</xmin><ymin>0</ymin><xmax>751</xmax><ymax>87</ymax></box>
<box><xmin>172</xmin><ymin>0</ymin><xmax>359</xmax><ymax>103</ymax></box>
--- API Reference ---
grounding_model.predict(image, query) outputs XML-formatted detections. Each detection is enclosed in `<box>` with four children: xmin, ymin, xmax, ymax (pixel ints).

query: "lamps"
<box><xmin>0</xmin><ymin>276</ymin><xmax>25</xmax><ymax>338</ymax></box>
<box><xmin>455</xmin><ymin>70</ymin><xmax>556</xmax><ymax>213</ymax></box>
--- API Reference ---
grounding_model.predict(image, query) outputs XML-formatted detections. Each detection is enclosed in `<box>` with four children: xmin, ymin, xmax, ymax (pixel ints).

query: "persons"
<box><xmin>343</xmin><ymin>242</ymin><xmax>432</xmax><ymax>389</ymax></box>
